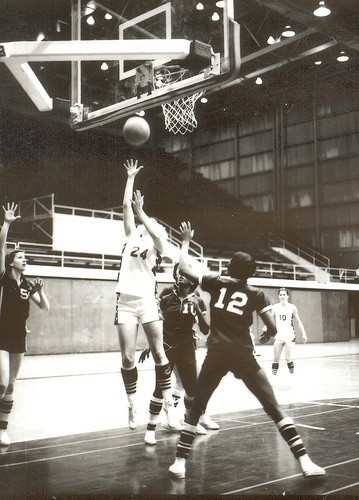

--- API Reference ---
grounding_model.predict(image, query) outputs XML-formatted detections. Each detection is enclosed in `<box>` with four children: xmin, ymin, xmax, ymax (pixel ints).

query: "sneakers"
<box><xmin>144</xmin><ymin>429</ymin><xmax>157</xmax><ymax>445</ymax></box>
<box><xmin>199</xmin><ymin>414</ymin><xmax>220</xmax><ymax>430</ymax></box>
<box><xmin>168</xmin><ymin>462</ymin><xmax>185</xmax><ymax>479</ymax></box>
<box><xmin>300</xmin><ymin>461</ymin><xmax>326</xmax><ymax>478</ymax></box>
<box><xmin>128</xmin><ymin>415</ymin><xmax>136</xmax><ymax>430</ymax></box>
<box><xmin>182</xmin><ymin>420</ymin><xmax>208</xmax><ymax>435</ymax></box>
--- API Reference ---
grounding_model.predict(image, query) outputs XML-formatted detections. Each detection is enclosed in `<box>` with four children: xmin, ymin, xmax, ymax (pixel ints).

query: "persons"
<box><xmin>169</xmin><ymin>220</ymin><xmax>326</xmax><ymax>478</ymax></box>
<box><xmin>262</xmin><ymin>288</ymin><xmax>307</xmax><ymax>375</ymax></box>
<box><xmin>0</xmin><ymin>202</ymin><xmax>49</xmax><ymax>446</ymax></box>
<box><xmin>138</xmin><ymin>263</ymin><xmax>219</xmax><ymax>444</ymax></box>
<box><xmin>114</xmin><ymin>159</ymin><xmax>179</xmax><ymax>430</ymax></box>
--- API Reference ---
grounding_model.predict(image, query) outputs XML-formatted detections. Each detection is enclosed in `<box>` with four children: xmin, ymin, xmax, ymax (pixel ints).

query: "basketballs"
<box><xmin>122</xmin><ymin>116</ymin><xmax>151</xmax><ymax>146</ymax></box>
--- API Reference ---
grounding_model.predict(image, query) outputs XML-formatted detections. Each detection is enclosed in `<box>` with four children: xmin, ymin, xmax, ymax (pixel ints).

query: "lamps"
<box><xmin>282</xmin><ymin>0</ymin><xmax>351</xmax><ymax>62</ymax></box>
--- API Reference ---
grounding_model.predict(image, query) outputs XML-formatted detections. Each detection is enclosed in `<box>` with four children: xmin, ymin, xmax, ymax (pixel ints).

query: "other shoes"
<box><xmin>0</xmin><ymin>429</ymin><xmax>9</xmax><ymax>445</ymax></box>
